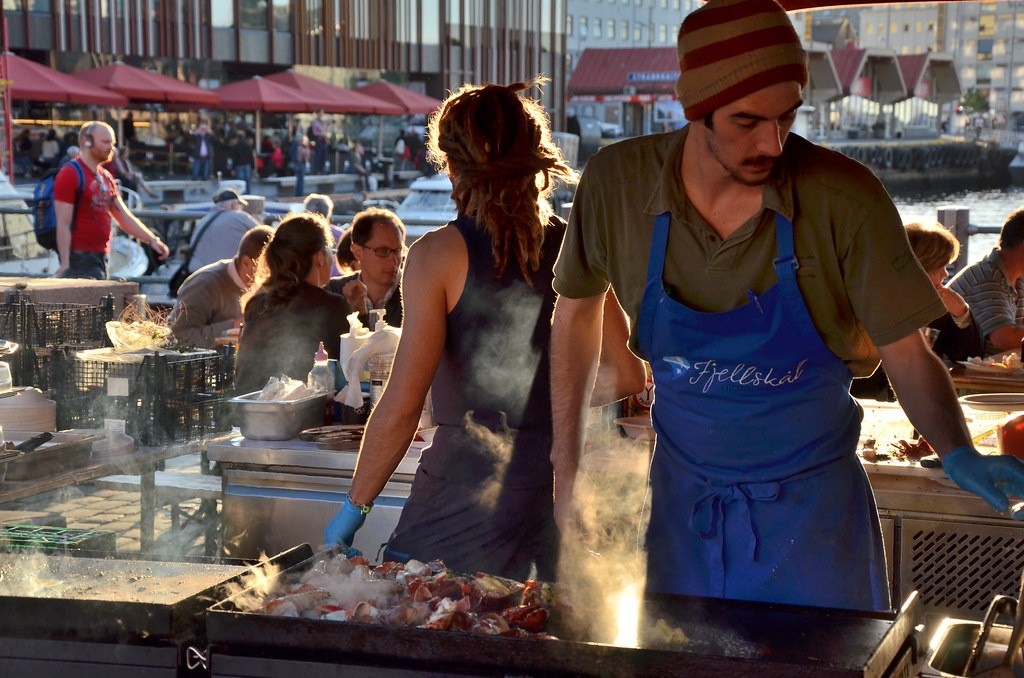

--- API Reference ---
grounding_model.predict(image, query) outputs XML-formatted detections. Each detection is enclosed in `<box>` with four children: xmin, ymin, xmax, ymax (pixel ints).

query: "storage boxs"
<box><xmin>0</xmin><ymin>296</ymin><xmax>238</xmax><ymax>442</ymax></box>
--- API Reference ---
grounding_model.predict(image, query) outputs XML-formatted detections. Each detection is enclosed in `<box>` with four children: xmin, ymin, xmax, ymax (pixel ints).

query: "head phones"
<box><xmin>83</xmin><ymin>120</ymin><xmax>98</xmax><ymax>148</ymax></box>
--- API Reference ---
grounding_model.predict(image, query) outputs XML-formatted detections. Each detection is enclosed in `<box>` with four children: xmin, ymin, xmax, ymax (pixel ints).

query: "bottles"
<box><xmin>307</xmin><ymin>342</ymin><xmax>334</xmax><ymax>391</ymax></box>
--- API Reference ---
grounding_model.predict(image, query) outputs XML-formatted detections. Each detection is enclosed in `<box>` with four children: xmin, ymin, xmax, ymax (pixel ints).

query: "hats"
<box><xmin>213</xmin><ymin>190</ymin><xmax>247</xmax><ymax>207</ymax></box>
<box><xmin>676</xmin><ymin>0</ymin><xmax>808</xmax><ymax>120</ymax></box>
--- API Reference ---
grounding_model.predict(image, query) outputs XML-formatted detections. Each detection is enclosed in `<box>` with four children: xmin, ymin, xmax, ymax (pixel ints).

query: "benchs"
<box><xmin>137</xmin><ymin>169</ymin><xmax>422</xmax><ymax>200</ymax></box>
<box><xmin>93</xmin><ymin>471</ymin><xmax>223</xmax><ymax>562</ymax></box>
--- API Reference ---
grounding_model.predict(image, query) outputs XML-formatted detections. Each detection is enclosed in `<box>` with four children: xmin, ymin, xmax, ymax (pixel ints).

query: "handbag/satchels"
<box><xmin>33</xmin><ymin>160</ymin><xmax>84</xmax><ymax>250</ymax></box>
<box><xmin>168</xmin><ymin>264</ymin><xmax>191</xmax><ymax>298</ymax></box>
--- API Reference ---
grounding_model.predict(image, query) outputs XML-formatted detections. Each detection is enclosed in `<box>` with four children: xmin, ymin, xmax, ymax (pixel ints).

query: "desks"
<box><xmin>132</xmin><ymin>208</ymin><xmax>207</xmax><ymax>257</ymax></box>
<box><xmin>0</xmin><ymin>431</ymin><xmax>244</xmax><ymax>561</ymax></box>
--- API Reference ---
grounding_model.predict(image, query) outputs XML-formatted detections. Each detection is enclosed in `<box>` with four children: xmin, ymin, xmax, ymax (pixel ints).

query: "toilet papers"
<box><xmin>338</xmin><ymin>310</ymin><xmax>377</xmax><ymax>382</ymax></box>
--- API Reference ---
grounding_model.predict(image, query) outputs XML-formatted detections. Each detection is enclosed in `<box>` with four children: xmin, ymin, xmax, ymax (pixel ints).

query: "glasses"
<box><xmin>93</xmin><ymin>173</ymin><xmax>107</xmax><ymax>194</ymax></box>
<box><xmin>359</xmin><ymin>245</ymin><xmax>402</xmax><ymax>257</ymax></box>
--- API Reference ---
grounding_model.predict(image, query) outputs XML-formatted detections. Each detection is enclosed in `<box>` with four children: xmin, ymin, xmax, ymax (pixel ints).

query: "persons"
<box><xmin>850</xmin><ymin>208</ymin><xmax>1024</xmax><ymax>404</ymax></box>
<box><xmin>974</xmin><ymin>114</ymin><xmax>983</xmax><ymax>138</ymax></box>
<box><xmin>548</xmin><ymin>0</ymin><xmax>1024</xmax><ymax>614</ymax></box>
<box><xmin>15</xmin><ymin>108</ymin><xmax>439</xmax><ymax>195</ymax></box>
<box><xmin>53</xmin><ymin>121</ymin><xmax>169</xmax><ymax>280</ymax></box>
<box><xmin>325</xmin><ymin>74</ymin><xmax>647</xmax><ymax>584</ymax></box>
<box><xmin>164</xmin><ymin>190</ymin><xmax>411</xmax><ymax>426</ymax></box>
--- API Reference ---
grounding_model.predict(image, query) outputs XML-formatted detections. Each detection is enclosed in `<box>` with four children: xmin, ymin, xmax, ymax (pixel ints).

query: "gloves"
<box><xmin>324</xmin><ymin>493</ymin><xmax>366</xmax><ymax>558</ymax></box>
<box><xmin>941</xmin><ymin>447</ymin><xmax>1024</xmax><ymax>520</ymax></box>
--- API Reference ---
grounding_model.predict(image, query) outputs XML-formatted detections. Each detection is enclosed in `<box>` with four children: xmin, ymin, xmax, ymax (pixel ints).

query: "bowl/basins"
<box><xmin>612</xmin><ymin>416</ymin><xmax>655</xmax><ymax>440</ymax></box>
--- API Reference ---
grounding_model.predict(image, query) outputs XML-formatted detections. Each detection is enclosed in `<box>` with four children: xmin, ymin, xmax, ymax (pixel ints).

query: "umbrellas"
<box><xmin>0</xmin><ymin>51</ymin><xmax>442</xmax><ymax>157</ymax></box>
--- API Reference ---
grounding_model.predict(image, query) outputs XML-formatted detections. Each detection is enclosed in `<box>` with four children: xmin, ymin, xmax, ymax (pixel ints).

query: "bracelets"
<box><xmin>347</xmin><ymin>488</ymin><xmax>374</xmax><ymax>513</ymax></box>
<box><xmin>150</xmin><ymin>236</ymin><xmax>161</xmax><ymax>247</ymax></box>
